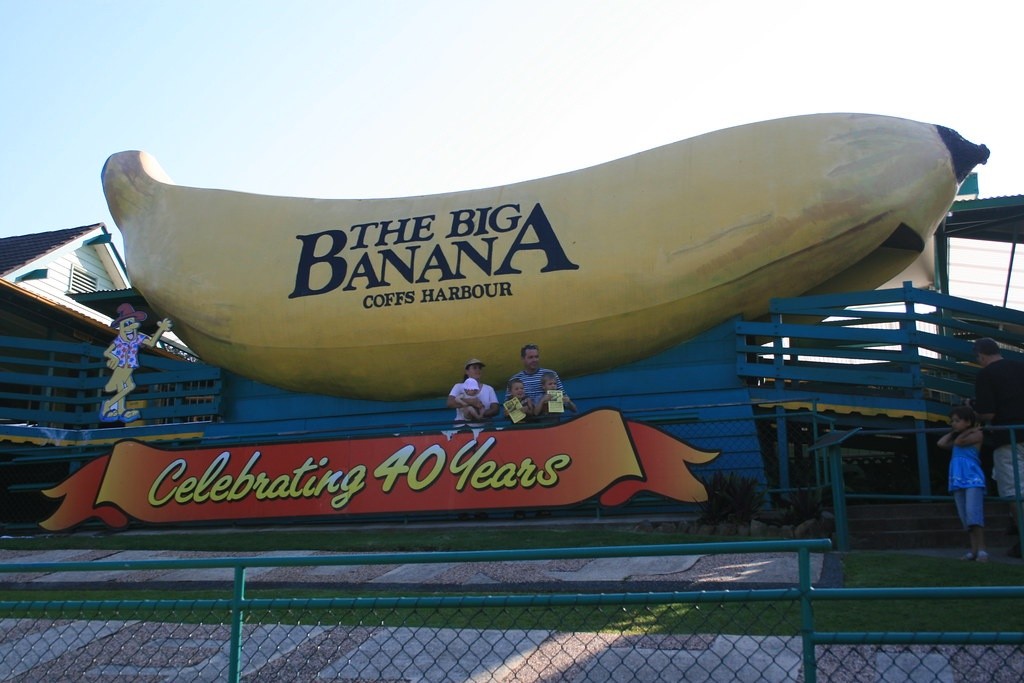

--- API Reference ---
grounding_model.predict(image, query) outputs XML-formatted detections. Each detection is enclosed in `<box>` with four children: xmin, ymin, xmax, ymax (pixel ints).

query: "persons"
<box><xmin>505</xmin><ymin>345</ymin><xmax>568</xmax><ymax>415</ymax></box>
<box><xmin>505</xmin><ymin>379</ymin><xmax>533</xmax><ymax>417</ymax></box>
<box><xmin>534</xmin><ymin>372</ymin><xmax>577</xmax><ymax>416</ymax></box>
<box><xmin>447</xmin><ymin>359</ymin><xmax>500</xmax><ymax>431</ymax></box>
<box><xmin>974</xmin><ymin>337</ymin><xmax>1024</xmax><ymax>559</ymax></box>
<box><xmin>937</xmin><ymin>407</ymin><xmax>990</xmax><ymax>562</ymax></box>
<box><xmin>455</xmin><ymin>378</ymin><xmax>485</xmax><ymax>419</ymax></box>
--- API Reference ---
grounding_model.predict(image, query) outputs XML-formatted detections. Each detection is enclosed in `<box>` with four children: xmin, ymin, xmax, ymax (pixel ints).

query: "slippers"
<box><xmin>976</xmin><ymin>550</ymin><xmax>992</xmax><ymax>563</ymax></box>
<box><xmin>961</xmin><ymin>552</ymin><xmax>978</xmax><ymax>561</ymax></box>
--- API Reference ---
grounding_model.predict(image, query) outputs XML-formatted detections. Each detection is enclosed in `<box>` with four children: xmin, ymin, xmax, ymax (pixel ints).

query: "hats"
<box><xmin>463</xmin><ymin>377</ymin><xmax>479</xmax><ymax>390</ymax></box>
<box><xmin>464</xmin><ymin>359</ymin><xmax>485</xmax><ymax>369</ymax></box>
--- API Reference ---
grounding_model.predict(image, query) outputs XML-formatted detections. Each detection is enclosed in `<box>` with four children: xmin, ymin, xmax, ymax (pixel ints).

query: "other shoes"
<box><xmin>514</xmin><ymin>510</ymin><xmax>525</xmax><ymax>520</ymax></box>
<box><xmin>1007</xmin><ymin>543</ymin><xmax>1022</xmax><ymax>558</ymax></box>
<box><xmin>455</xmin><ymin>512</ymin><xmax>469</xmax><ymax>521</ymax></box>
<box><xmin>1007</xmin><ymin>525</ymin><xmax>1019</xmax><ymax>535</ymax></box>
<box><xmin>536</xmin><ymin>511</ymin><xmax>552</xmax><ymax>518</ymax></box>
<box><xmin>479</xmin><ymin>511</ymin><xmax>488</xmax><ymax>521</ymax></box>
